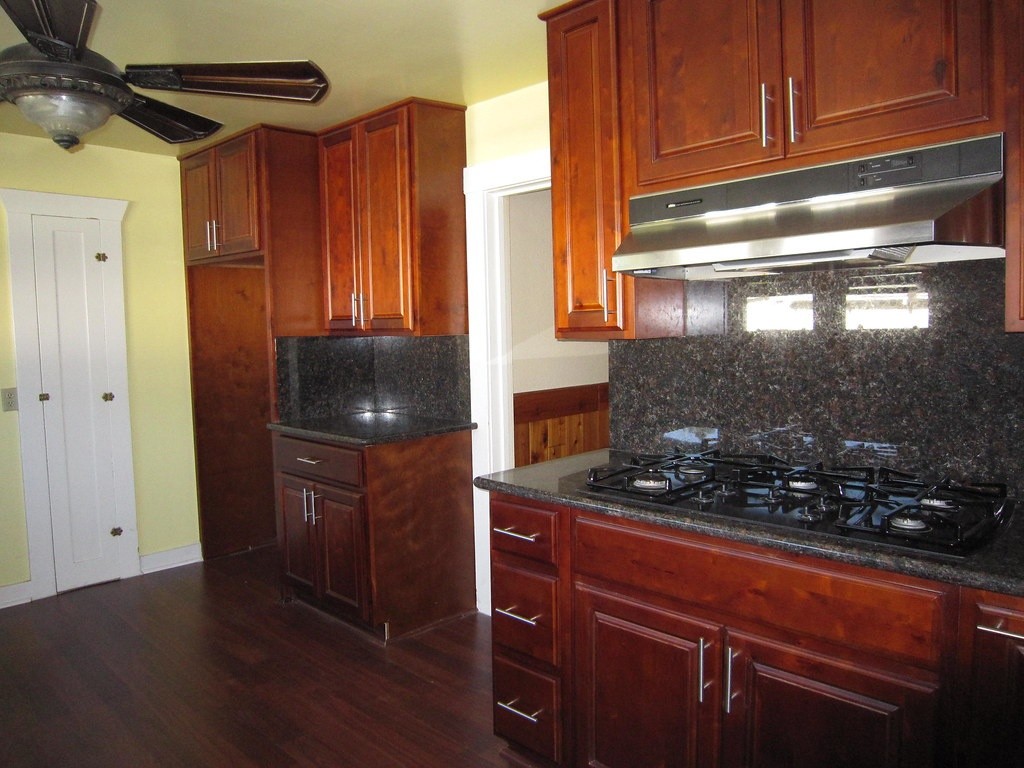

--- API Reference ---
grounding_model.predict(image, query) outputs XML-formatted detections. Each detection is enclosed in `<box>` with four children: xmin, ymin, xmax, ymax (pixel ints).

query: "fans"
<box><xmin>1</xmin><ymin>0</ymin><xmax>330</xmax><ymax>151</ymax></box>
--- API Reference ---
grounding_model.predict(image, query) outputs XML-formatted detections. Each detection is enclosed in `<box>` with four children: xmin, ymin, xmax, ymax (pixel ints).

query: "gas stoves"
<box><xmin>574</xmin><ymin>444</ymin><xmax>1012</xmax><ymax>562</ymax></box>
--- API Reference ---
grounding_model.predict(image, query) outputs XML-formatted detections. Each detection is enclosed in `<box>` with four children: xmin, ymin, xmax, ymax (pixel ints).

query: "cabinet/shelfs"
<box><xmin>958</xmin><ymin>583</ymin><xmax>1024</xmax><ymax>768</ymax></box>
<box><xmin>271</xmin><ymin>431</ymin><xmax>476</xmax><ymax>644</ymax></box>
<box><xmin>537</xmin><ymin>0</ymin><xmax>687</xmax><ymax>341</ymax></box>
<box><xmin>569</xmin><ymin>507</ymin><xmax>959</xmax><ymax>767</ymax></box>
<box><xmin>174</xmin><ymin>123</ymin><xmax>314</xmax><ymax>267</ymax></box>
<box><xmin>488</xmin><ymin>489</ymin><xmax>570</xmax><ymax>768</ymax></box>
<box><xmin>316</xmin><ymin>95</ymin><xmax>469</xmax><ymax>338</ymax></box>
<box><xmin>1003</xmin><ymin>1</ymin><xmax>1024</xmax><ymax>333</ymax></box>
<box><xmin>622</xmin><ymin>0</ymin><xmax>1005</xmax><ymax>200</ymax></box>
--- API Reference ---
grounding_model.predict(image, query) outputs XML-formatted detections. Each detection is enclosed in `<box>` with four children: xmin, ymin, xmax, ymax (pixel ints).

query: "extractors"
<box><xmin>611</xmin><ymin>133</ymin><xmax>1005</xmax><ymax>282</ymax></box>
